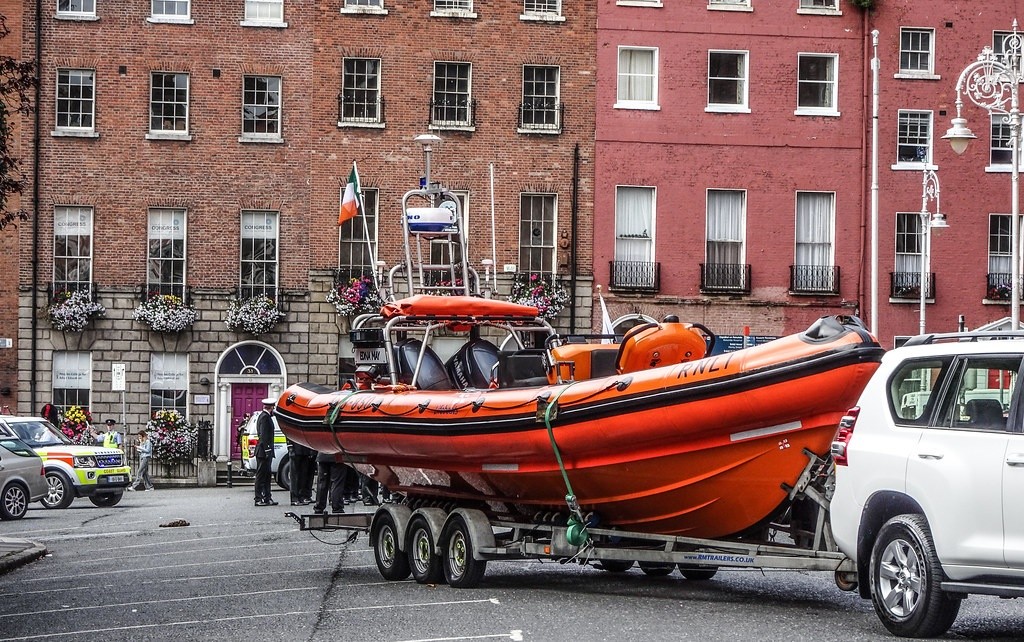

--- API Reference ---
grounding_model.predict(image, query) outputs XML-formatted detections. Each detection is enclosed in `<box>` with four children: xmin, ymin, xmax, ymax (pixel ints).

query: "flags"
<box><xmin>601</xmin><ymin>294</ymin><xmax>615</xmax><ymax>344</ymax></box>
<box><xmin>339</xmin><ymin>167</ymin><xmax>361</xmax><ymax>225</ymax></box>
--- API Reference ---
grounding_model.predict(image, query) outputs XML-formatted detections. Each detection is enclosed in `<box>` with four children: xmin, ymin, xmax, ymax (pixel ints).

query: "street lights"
<box><xmin>939</xmin><ymin>19</ymin><xmax>1024</xmax><ymax>410</ymax></box>
<box><xmin>916</xmin><ymin>155</ymin><xmax>950</xmax><ymax>392</ymax></box>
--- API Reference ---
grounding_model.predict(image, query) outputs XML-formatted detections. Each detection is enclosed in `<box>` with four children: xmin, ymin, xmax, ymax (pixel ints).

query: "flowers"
<box><xmin>140</xmin><ymin>406</ymin><xmax>203</xmax><ymax>465</ymax></box>
<box><xmin>55</xmin><ymin>403</ymin><xmax>95</xmax><ymax>446</ymax></box>
<box><xmin>323</xmin><ymin>275</ymin><xmax>382</xmax><ymax>319</ymax></box>
<box><xmin>507</xmin><ymin>274</ymin><xmax>579</xmax><ymax>328</ymax></box>
<box><xmin>224</xmin><ymin>294</ymin><xmax>285</xmax><ymax>334</ymax></box>
<box><xmin>419</xmin><ymin>273</ymin><xmax>482</xmax><ymax>330</ymax></box>
<box><xmin>43</xmin><ymin>288</ymin><xmax>104</xmax><ymax>332</ymax></box>
<box><xmin>132</xmin><ymin>294</ymin><xmax>200</xmax><ymax>332</ymax></box>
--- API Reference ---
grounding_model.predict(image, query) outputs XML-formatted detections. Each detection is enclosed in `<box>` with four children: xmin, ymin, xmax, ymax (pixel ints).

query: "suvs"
<box><xmin>0</xmin><ymin>412</ymin><xmax>133</xmax><ymax>510</ymax></box>
<box><xmin>240</xmin><ymin>410</ymin><xmax>319</xmax><ymax>491</ymax></box>
<box><xmin>827</xmin><ymin>331</ymin><xmax>1024</xmax><ymax>638</ymax></box>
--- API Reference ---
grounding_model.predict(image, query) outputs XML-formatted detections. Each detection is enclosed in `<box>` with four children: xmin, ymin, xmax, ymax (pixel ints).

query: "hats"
<box><xmin>106</xmin><ymin>419</ymin><xmax>116</xmax><ymax>426</ymax></box>
<box><xmin>262</xmin><ymin>397</ymin><xmax>277</xmax><ymax>406</ymax></box>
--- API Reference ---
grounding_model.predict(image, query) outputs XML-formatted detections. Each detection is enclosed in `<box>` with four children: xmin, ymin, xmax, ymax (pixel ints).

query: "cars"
<box><xmin>0</xmin><ymin>430</ymin><xmax>49</xmax><ymax>521</ymax></box>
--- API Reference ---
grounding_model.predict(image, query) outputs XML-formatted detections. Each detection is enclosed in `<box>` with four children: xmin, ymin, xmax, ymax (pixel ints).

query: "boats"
<box><xmin>274</xmin><ymin>132</ymin><xmax>890</xmax><ymax>551</ymax></box>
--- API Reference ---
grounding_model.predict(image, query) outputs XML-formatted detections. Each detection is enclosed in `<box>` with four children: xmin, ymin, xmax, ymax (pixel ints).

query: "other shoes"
<box><xmin>145</xmin><ymin>488</ymin><xmax>154</xmax><ymax>492</ymax></box>
<box><xmin>129</xmin><ymin>486</ymin><xmax>135</xmax><ymax>491</ymax></box>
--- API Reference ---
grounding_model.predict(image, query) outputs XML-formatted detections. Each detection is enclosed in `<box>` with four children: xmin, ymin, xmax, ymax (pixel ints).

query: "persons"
<box><xmin>127</xmin><ymin>430</ymin><xmax>154</xmax><ymax>491</ymax></box>
<box><xmin>286</xmin><ymin>437</ymin><xmax>391</xmax><ymax>514</ymax></box>
<box><xmin>89</xmin><ymin>419</ymin><xmax>122</xmax><ymax>449</ymax></box>
<box><xmin>254</xmin><ymin>398</ymin><xmax>278</xmax><ymax>506</ymax></box>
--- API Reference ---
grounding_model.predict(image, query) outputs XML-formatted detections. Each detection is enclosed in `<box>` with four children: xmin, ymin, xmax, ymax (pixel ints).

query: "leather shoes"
<box><xmin>303</xmin><ymin>498</ymin><xmax>311</xmax><ymax>504</ymax></box>
<box><xmin>328</xmin><ymin>496</ymin><xmax>392</xmax><ymax>506</ymax></box>
<box><xmin>254</xmin><ymin>499</ymin><xmax>269</xmax><ymax>506</ymax></box>
<box><xmin>265</xmin><ymin>499</ymin><xmax>279</xmax><ymax>505</ymax></box>
<box><xmin>310</xmin><ymin>499</ymin><xmax>316</xmax><ymax>503</ymax></box>
<box><xmin>291</xmin><ymin>501</ymin><xmax>304</xmax><ymax>506</ymax></box>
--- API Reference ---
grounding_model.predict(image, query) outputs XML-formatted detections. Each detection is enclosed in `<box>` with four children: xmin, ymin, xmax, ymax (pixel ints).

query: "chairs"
<box><xmin>964</xmin><ymin>399</ymin><xmax>1006</xmax><ymax>427</ymax></box>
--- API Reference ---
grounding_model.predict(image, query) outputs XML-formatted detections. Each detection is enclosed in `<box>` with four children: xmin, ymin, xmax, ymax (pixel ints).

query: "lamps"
<box><xmin>200</xmin><ymin>377</ymin><xmax>210</xmax><ymax>386</ymax></box>
